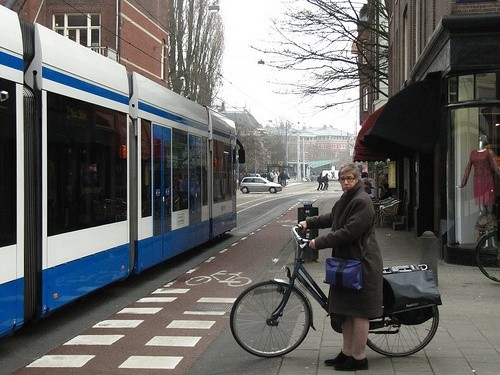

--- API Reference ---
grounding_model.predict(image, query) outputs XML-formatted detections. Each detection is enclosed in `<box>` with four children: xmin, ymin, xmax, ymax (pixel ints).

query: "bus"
<box><xmin>0</xmin><ymin>5</ymin><xmax>246</xmax><ymax>338</ymax></box>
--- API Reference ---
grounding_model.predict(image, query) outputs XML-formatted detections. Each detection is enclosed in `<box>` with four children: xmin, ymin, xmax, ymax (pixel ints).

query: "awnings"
<box><xmin>353</xmin><ymin>80</ymin><xmax>438</xmax><ymax>163</ymax></box>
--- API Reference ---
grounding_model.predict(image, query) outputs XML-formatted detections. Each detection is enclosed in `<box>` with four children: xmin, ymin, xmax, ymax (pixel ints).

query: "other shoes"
<box><xmin>324</xmin><ymin>351</ymin><xmax>369</xmax><ymax>371</ymax></box>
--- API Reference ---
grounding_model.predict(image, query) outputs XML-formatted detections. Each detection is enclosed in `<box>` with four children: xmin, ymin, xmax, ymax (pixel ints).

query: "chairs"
<box><xmin>373</xmin><ymin>196</ymin><xmax>400</xmax><ymax>229</ymax></box>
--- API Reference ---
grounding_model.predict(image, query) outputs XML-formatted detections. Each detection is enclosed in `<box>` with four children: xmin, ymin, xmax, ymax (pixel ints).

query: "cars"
<box><xmin>240</xmin><ymin>177</ymin><xmax>282</xmax><ymax>194</ymax></box>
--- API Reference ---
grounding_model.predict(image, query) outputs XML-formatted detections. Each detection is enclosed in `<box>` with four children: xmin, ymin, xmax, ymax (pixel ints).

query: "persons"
<box><xmin>380</xmin><ymin>174</ymin><xmax>388</xmax><ymax>200</ymax></box>
<box><xmin>459</xmin><ymin>135</ymin><xmax>500</xmax><ymax>248</ymax></box>
<box><xmin>317</xmin><ymin>173</ymin><xmax>323</xmax><ymax>191</ymax></box>
<box><xmin>361</xmin><ymin>172</ymin><xmax>372</xmax><ymax>194</ymax></box>
<box><xmin>299</xmin><ymin>164</ymin><xmax>383</xmax><ymax>371</ymax></box>
<box><xmin>234</xmin><ymin>169</ymin><xmax>290</xmax><ymax>190</ymax></box>
<box><xmin>323</xmin><ymin>174</ymin><xmax>329</xmax><ymax>191</ymax></box>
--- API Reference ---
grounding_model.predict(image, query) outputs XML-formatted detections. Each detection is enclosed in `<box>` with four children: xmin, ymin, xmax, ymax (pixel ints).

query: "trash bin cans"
<box><xmin>298</xmin><ymin>199</ymin><xmax>318</xmax><ymax>262</ymax></box>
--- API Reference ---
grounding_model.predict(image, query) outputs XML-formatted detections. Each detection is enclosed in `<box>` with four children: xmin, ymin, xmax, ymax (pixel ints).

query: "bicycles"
<box><xmin>474</xmin><ymin>228</ymin><xmax>500</xmax><ymax>282</ymax></box>
<box><xmin>230</xmin><ymin>224</ymin><xmax>439</xmax><ymax>357</ymax></box>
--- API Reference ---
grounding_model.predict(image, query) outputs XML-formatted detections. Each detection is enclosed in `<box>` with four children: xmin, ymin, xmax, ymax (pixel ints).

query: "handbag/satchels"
<box><xmin>383</xmin><ymin>269</ymin><xmax>442</xmax><ymax>325</ymax></box>
<box><xmin>322</xmin><ymin>256</ymin><xmax>363</xmax><ymax>290</ymax></box>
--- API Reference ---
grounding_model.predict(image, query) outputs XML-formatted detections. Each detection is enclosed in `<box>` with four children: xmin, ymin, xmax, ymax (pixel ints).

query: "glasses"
<box><xmin>338</xmin><ymin>178</ymin><xmax>355</xmax><ymax>183</ymax></box>
